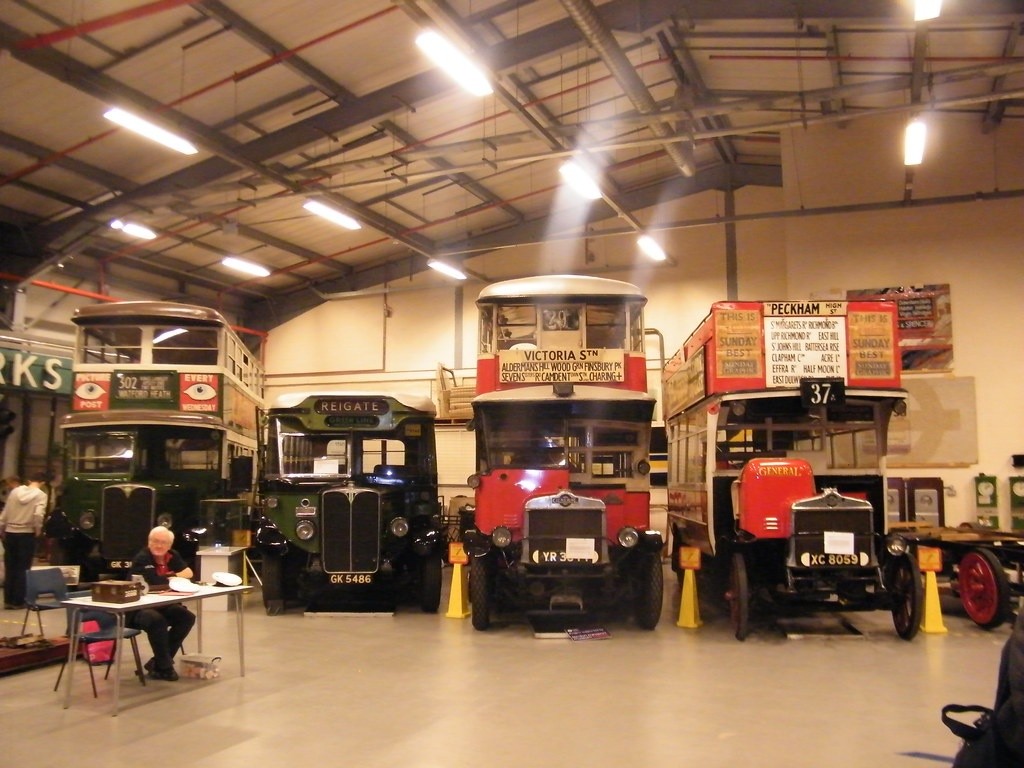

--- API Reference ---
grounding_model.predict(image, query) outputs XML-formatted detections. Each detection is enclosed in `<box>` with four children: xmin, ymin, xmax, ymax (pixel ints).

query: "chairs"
<box><xmin>51</xmin><ymin>586</ymin><xmax>148</xmax><ymax>698</ymax></box>
<box><xmin>21</xmin><ymin>566</ymin><xmax>72</xmax><ymax>640</ymax></box>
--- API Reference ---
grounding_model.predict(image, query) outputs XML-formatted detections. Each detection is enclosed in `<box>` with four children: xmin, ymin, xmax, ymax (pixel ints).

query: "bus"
<box><xmin>658</xmin><ymin>293</ymin><xmax>918</xmax><ymax>644</ymax></box>
<box><xmin>461</xmin><ymin>277</ymin><xmax>666</xmax><ymax>631</ymax></box>
<box><xmin>257</xmin><ymin>389</ymin><xmax>446</xmax><ymax>615</ymax></box>
<box><xmin>53</xmin><ymin>297</ymin><xmax>267</xmax><ymax>579</ymax></box>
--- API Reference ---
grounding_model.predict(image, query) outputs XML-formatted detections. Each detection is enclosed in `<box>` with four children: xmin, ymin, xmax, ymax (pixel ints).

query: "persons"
<box><xmin>123</xmin><ymin>526</ymin><xmax>195</xmax><ymax>679</ymax></box>
<box><xmin>2</xmin><ymin>470</ymin><xmax>50</xmax><ymax>604</ymax></box>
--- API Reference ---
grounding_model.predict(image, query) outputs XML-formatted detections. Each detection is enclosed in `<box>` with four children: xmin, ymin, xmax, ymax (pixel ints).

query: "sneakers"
<box><xmin>144</xmin><ymin>657</ymin><xmax>178</xmax><ymax>681</ymax></box>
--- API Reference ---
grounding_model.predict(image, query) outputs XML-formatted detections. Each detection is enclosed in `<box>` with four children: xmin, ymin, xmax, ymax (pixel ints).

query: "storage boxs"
<box><xmin>91</xmin><ymin>580</ymin><xmax>142</xmax><ymax>604</ymax></box>
<box><xmin>179</xmin><ymin>651</ymin><xmax>222</xmax><ymax>680</ymax></box>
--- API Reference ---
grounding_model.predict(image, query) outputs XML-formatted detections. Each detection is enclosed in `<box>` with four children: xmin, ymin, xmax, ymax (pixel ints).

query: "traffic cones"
<box><xmin>444</xmin><ymin>562</ymin><xmax>472</xmax><ymax>619</ymax></box>
<box><xmin>674</xmin><ymin>568</ymin><xmax>704</xmax><ymax>630</ymax></box>
<box><xmin>919</xmin><ymin>570</ymin><xmax>951</xmax><ymax>636</ymax></box>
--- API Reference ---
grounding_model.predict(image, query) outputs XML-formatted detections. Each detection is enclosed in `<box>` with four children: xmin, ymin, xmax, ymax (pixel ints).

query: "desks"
<box><xmin>195</xmin><ymin>545</ymin><xmax>251</xmax><ymax>613</ymax></box>
<box><xmin>60</xmin><ymin>579</ymin><xmax>255</xmax><ymax>718</ymax></box>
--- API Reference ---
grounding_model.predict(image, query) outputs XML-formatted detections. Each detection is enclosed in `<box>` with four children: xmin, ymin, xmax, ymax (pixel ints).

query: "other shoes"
<box><xmin>4</xmin><ymin>603</ymin><xmax>27</xmax><ymax>609</ymax></box>
